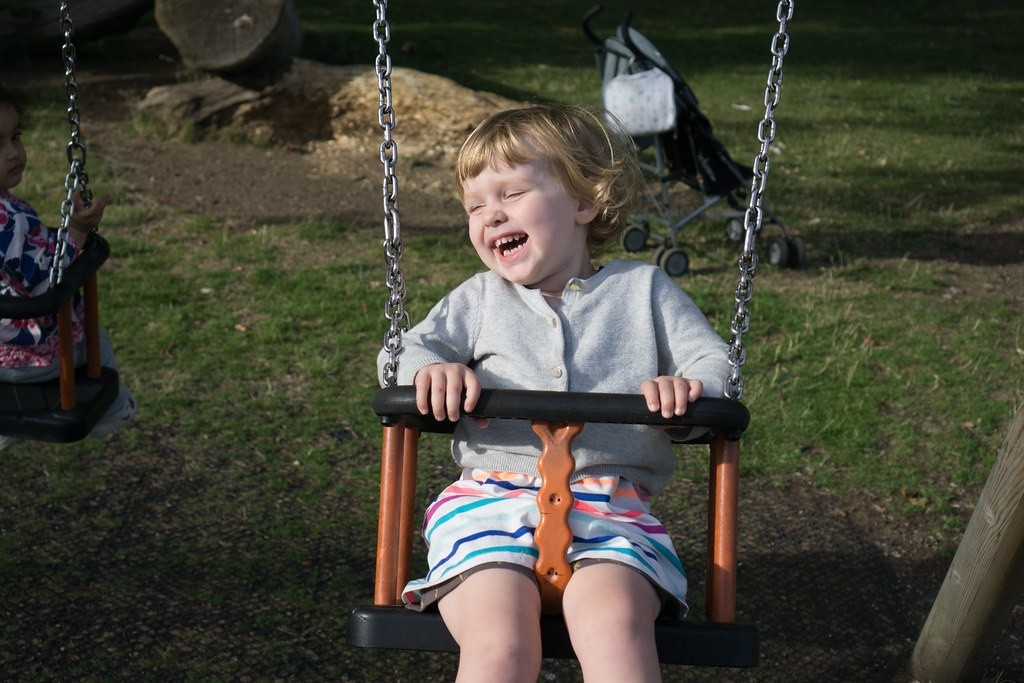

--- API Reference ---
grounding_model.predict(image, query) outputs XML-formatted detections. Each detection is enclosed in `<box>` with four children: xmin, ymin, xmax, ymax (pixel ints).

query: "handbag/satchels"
<box><xmin>603</xmin><ymin>56</ymin><xmax>677</xmax><ymax>135</ymax></box>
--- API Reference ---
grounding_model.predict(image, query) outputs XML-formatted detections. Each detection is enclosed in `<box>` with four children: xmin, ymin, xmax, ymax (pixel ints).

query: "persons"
<box><xmin>377</xmin><ymin>108</ymin><xmax>733</xmax><ymax>683</ymax></box>
<box><xmin>0</xmin><ymin>84</ymin><xmax>137</xmax><ymax>437</ymax></box>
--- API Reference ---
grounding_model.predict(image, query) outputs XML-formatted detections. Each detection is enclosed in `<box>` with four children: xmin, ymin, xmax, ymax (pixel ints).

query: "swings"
<box><xmin>1</xmin><ymin>2</ymin><xmax>120</xmax><ymax>446</ymax></box>
<box><xmin>339</xmin><ymin>0</ymin><xmax>794</xmax><ymax>670</ymax></box>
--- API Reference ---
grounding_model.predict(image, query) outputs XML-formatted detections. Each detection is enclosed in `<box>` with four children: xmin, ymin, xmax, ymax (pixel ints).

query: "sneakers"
<box><xmin>0</xmin><ymin>435</ymin><xmax>18</xmax><ymax>450</ymax></box>
<box><xmin>91</xmin><ymin>394</ymin><xmax>137</xmax><ymax>435</ymax></box>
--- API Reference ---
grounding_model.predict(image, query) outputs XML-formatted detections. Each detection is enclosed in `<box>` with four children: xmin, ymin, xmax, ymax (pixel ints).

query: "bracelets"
<box><xmin>70</xmin><ymin>216</ymin><xmax>98</xmax><ymax>233</ymax></box>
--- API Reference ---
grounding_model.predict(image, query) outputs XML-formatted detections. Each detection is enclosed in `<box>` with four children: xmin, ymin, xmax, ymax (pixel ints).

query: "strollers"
<box><xmin>582</xmin><ymin>5</ymin><xmax>805</xmax><ymax>279</ymax></box>
